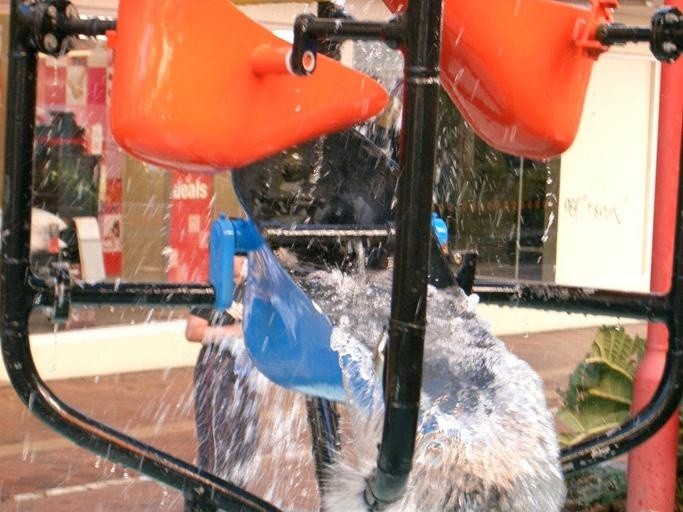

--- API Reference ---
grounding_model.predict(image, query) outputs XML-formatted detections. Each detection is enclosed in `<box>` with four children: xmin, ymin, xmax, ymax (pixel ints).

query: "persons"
<box><xmin>184</xmin><ymin>253</ymin><xmax>264</xmax><ymax>512</ymax></box>
